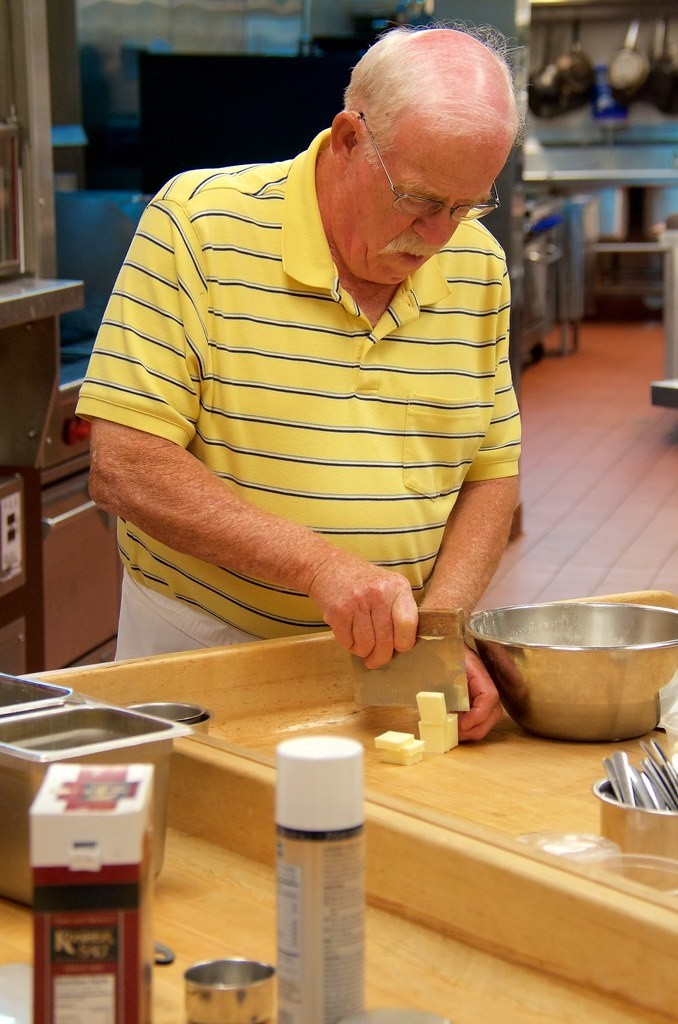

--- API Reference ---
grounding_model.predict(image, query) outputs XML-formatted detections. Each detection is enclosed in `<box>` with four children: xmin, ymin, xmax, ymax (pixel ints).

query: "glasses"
<box><xmin>356</xmin><ymin>112</ymin><xmax>501</xmax><ymax>224</ymax></box>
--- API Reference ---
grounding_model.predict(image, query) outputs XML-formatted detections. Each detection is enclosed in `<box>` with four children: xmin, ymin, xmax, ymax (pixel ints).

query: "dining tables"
<box><xmin>0</xmin><ymin>586</ymin><xmax>678</xmax><ymax>1024</ymax></box>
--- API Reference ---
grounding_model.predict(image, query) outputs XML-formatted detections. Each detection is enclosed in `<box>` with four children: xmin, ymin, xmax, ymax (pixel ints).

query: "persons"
<box><xmin>73</xmin><ymin>27</ymin><xmax>524</xmax><ymax>741</ymax></box>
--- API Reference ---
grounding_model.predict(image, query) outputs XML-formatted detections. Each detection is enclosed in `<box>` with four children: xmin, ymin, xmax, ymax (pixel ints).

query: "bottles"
<box><xmin>277</xmin><ymin>735</ymin><xmax>365</xmax><ymax>1024</ymax></box>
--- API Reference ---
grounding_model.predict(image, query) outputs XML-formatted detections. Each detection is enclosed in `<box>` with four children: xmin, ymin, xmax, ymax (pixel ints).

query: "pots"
<box><xmin>529</xmin><ymin>19</ymin><xmax>593</xmax><ymax>118</ymax></box>
<box><xmin>609</xmin><ymin>12</ymin><xmax>677</xmax><ymax>115</ymax></box>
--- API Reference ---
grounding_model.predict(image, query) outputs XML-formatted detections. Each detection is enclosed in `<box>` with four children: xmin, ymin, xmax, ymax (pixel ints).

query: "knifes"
<box><xmin>350</xmin><ymin>607</ymin><xmax>470</xmax><ymax>711</ymax></box>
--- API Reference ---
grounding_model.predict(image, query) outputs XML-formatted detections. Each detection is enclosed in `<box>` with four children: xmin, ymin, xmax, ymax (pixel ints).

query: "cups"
<box><xmin>592</xmin><ymin>769</ymin><xmax>677</xmax><ymax>887</ymax></box>
<box><xmin>126</xmin><ymin>703</ymin><xmax>214</xmax><ymax>734</ymax></box>
<box><xmin>183</xmin><ymin>959</ymin><xmax>276</xmax><ymax>1024</ymax></box>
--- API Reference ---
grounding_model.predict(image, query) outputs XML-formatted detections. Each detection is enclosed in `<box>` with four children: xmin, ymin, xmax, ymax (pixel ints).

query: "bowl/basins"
<box><xmin>465</xmin><ymin>602</ymin><xmax>677</xmax><ymax>741</ymax></box>
